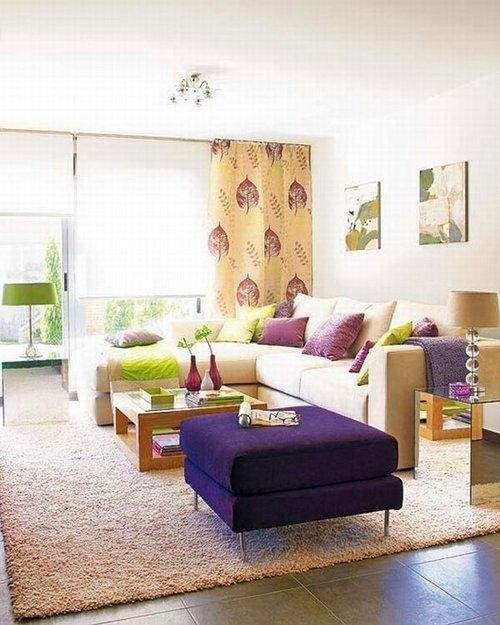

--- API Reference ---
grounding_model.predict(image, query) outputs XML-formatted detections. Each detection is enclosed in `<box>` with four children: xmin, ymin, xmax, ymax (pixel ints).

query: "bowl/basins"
<box><xmin>449</xmin><ymin>382</ymin><xmax>471</xmax><ymax>396</ymax></box>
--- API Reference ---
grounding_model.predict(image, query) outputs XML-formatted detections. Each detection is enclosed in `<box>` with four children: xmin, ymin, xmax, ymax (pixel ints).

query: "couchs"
<box><xmin>76</xmin><ymin>293</ymin><xmax>499</xmax><ymax>472</ymax></box>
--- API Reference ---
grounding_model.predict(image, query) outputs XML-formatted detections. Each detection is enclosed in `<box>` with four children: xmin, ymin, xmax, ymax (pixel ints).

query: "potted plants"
<box><xmin>177</xmin><ymin>337</ymin><xmax>202</xmax><ymax>392</ymax></box>
<box><xmin>195</xmin><ymin>324</ymin><xmax>223</xmax><ymax>391</ymax></box>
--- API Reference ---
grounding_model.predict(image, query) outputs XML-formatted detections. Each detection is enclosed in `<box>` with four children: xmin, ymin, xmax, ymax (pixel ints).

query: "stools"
<box><xmin>179</xmin><ymin>404</ymin><xmax>405</xmax><ymax>560</ymax></box>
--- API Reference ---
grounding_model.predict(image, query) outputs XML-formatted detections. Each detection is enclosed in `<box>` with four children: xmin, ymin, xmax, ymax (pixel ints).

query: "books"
<box><xmin>152</xmin><ymin>426</ymin><xmax>184</xmax><ymax>456</ymax></box>
<box><xmin>238</xmin><ymin>402</ymin><xmax>300</xmax><ymax>428</ymax></box>
<box><xmin>186</xmin><ymin>390</ymin><xmax>244</xmax><ymax>399</ymax></box>
<box><xmin>185</xmin><ymin>390</ymin><xmax>244</xmax><ymax>403</ymax></box>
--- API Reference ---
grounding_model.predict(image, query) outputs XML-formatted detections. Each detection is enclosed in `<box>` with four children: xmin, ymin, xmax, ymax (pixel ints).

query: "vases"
<box><xmin>199</xmin><ymin>371</ymin><xmax>215</xmax><ymax>391</ymax></box>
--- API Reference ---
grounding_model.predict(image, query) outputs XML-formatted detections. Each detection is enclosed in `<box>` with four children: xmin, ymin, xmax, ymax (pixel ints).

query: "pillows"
<box><xmin>348</xmin><ymin>317</ymin><xmax>440</xmax><ymax>386</ymax></box>
<box><xmin>302</xmin><ymin>309</ymin><xmax>366</xmax><ymax>361</ymax></box>
<box><xmin>236</xmin><ymin>302</ymin><xmax>277</xmax><ymax>341</ymax></box>
<box><xmin>105</xmin><ymin>327</ymin><xmax>163</xmax><ymax>349</ymax></box>
<box><xmin>260</xmin><ymin>316</ymin><xmax>310</xmax><ymax>349</ymax></box>
<box><xmin>275</xmin><ymin>298</ymin><xmax>296</xmax><ymax>317</ymax></box>
<box><xmin>216</xmin><ymin>316</ymin><xmax>259</xmax><ymax>344</ymax></box>
<box><xmin>172</xmin><ymin>317</ymin><xmax>225</xmax><ymax>344</ymax></box>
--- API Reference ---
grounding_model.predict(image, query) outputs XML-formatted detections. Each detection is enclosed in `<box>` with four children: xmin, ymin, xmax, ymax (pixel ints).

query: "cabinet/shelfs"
<box><xmin>411</xmin><ymin>387</ymin><xmax>500</xmax><ymax>506</ymax></box>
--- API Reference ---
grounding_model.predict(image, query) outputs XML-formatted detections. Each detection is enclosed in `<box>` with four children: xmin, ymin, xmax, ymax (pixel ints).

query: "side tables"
<box><xmin>415</xmin><ymin>393</ymin><xmax>485</xmax><ymax>441</ymax></box>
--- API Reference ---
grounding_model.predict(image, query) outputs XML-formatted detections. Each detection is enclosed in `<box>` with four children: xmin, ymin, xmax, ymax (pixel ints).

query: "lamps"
<box><xmin>3</xmin><ymin>281</ymin><xmax>60</xmax><ymax>356</ymax></box>
<box><xmin>166</xmin><ymin>66</ymin><xmax>218</xmax><ymax>106</ymax></box>
<box><xmin>442</xmin><ymin>289</ymin><xmax>498</xmax><ymax>394</ymax></box>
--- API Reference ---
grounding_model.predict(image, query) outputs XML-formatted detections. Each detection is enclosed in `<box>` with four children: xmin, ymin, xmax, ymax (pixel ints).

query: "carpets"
<box><xmin>412</xmin><ymin>441</ymin><xmax>500</xmax><ymax>506</ymax></box>
<box><xmin>1</xmin><ymin>400</ymin><xmax>499</xmax><ymax>621</ymax></box>
<box><xmin>3</xmin><ymin>366</ymin><xmax>72</xmax><ymax>424</ymax></box>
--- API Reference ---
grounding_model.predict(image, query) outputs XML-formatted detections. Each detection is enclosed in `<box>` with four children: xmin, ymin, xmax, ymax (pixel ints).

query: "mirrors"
<box><xmin>412</xmin><ymin>393</ymin><xmax>470</xmax><ymax>504</ymax></box>
<box><xmin>470</xmin><ymin>401</ymin><xmax>499</xmax><ymax>505</ymax></box>
